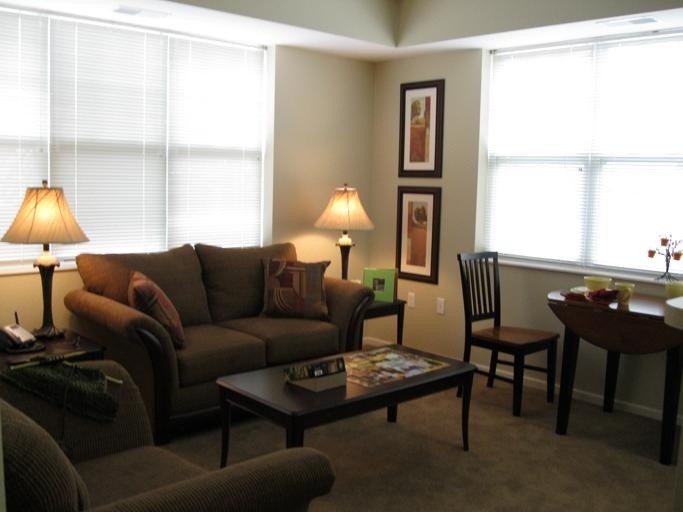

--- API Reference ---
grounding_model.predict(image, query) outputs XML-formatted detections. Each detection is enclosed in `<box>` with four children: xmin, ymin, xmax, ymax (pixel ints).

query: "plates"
<box><xmin>570</xmin><ymin>285</ymin><xmax>611</xmax><ymax>295</ymax></box>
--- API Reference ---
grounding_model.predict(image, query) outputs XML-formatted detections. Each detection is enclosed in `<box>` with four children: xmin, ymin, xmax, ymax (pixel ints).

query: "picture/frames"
<box><xmin>397</xmin><ymin>78</ymin><xmax>444</xmax><ymax>179</ymax></box>
<box><xmin>392</xmin><ymin>185</ymin><xmax>443</xmax><ymax>285</ymax></box>
<box><xmin>361</xmin><ymin>269</ymin><xmax>395</xmax><ymax>303</ymax></box>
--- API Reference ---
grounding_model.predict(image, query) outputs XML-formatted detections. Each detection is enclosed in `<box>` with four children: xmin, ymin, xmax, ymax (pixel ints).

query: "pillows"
<box><xmin>259</xmin><ymin>257</ymin><xmax>333</xmax><ymax>322</ymax></box>
<box><xmin>125</xmin><ymin>269</ymin><xmax>185</xmax><ymax>350</ymax></box>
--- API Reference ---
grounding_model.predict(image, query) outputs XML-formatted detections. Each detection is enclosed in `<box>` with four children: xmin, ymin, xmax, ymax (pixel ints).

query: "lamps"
<box><xmin>313</xmin><ymin>183</ymin><xmax>374</xmax><ymax>281</ymax></box>
<box><xmin>0</xmin><ymin>181</ymin><xmax>91</xmax><ymax>339</ymax></box>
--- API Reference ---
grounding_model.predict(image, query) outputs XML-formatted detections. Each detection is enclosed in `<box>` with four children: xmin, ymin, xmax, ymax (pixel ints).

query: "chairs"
<box><xmin>456</xmin><ymin>251</ymin><xmax>560</xmax><ymax>418</ymax></box>
<box><xmin>0</xmin><ymin>360</ymin><xmax>335</xmax><ymax>512</ymax></box>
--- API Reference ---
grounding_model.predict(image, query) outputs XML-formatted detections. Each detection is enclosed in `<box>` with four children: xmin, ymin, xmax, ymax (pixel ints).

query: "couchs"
<box><xmin>64</xmin><ymin>242</ymin><xmax>378</xmax><ymax>432</ymax></box>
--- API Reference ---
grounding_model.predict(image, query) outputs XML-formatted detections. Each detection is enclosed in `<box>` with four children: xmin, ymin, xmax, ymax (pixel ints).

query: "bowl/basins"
<box><xmin>583</xmin><ymin>276</ymin><xmax>611</xmax><ymax>291</ymax></box>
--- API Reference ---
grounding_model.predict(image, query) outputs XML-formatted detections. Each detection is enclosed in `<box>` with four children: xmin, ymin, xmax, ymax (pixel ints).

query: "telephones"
<box><xmin>0</xmin><ymin>312</ymin><xmax>36</xmax><ymax>349</ymax></box>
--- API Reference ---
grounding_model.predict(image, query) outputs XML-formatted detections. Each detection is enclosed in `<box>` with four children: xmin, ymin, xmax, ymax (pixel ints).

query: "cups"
<box><xmin>665</xmin><ymin>282</ymin><xmax>683</xmax><ymax>298</ymax></box>
<box><xmin>614</xmin><ymin>282</ymin><xmax>635</xmax><ymax>304</ymax></box>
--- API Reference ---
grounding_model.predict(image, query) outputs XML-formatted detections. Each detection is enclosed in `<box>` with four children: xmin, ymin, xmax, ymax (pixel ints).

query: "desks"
<box><xmin>545</xmin><ymin>286</ymin><xmax>682</xmax><ymax>467</ymax></box>
<box><xmin>0</xmin><ymin>328</ymin><xmax>106</xmax><ymax>371</ymax></box>
<box><xmin>361</xmin><ymin>298</ymin><xmax>407</xmax><ymax>345</ymax></box>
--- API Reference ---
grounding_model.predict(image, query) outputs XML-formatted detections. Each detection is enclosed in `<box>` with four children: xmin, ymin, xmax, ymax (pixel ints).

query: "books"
<box><xmin>361</xmin><ymin>267</ymin><xmax>398</xmax><ymax>306</ymax></box>
